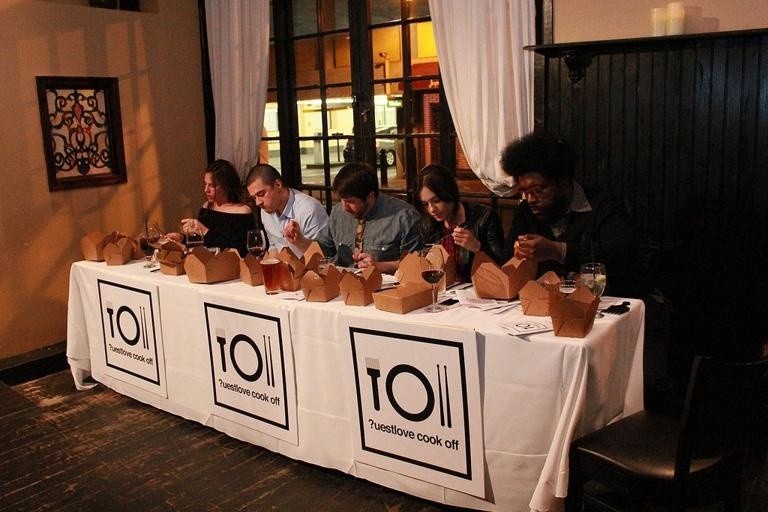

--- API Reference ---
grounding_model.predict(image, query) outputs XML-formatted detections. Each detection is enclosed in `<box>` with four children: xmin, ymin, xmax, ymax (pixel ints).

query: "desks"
<box><xmin>72</xmin><ymin>256</ymin><xmax>645</xmax><ymax>512</ymax></box>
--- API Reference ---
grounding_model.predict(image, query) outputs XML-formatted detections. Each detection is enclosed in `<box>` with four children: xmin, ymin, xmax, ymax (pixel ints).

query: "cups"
<box><xmin>185</xmin><ymin>226</ymin><xmax>204</xmax><ymax>251</ymax></box>
<box><xmin>259</xmin><ymin>258</ymin><xmax>281</xmax><ymax>296</ymax></box>
<box><xmin>318</xmin><ymin>256</ymin><xmax>335</xmax><ymax>276</ymax></box>
<box><xmin>246</xmin><ymin>228</ymin><xmax>267</xmax><ymax>260</ymax></box>
<box><xmin>558</xmin><ymin>279</ymin><xmax>577</xmax><ymax>294</ymax></box>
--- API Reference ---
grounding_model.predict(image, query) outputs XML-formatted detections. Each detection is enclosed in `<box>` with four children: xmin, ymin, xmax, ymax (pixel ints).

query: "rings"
<box><xmin>188</xmin><ymin>221</ymin><xmax>193</xmax><ymax>228</ymax></box>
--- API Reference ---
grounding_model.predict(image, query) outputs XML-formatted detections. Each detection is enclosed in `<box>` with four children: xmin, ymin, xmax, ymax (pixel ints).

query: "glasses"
<box><xmin>513</xmin><ymin>177</ymin><xmax>554</xmax><ymax>202</ymax></box>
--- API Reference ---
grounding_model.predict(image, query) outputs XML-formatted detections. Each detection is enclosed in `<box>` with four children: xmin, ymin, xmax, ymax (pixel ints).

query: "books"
<box><xmin>334</xmin><ymin>265</ymin><xmax>399</xmax><ymax>290</ymax></box>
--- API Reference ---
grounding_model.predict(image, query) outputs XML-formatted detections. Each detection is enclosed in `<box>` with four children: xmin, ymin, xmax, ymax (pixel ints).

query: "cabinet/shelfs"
<box><xmin>518</xmin><ymin>27</ymin><xmax>768</xmax><ymax>473</ymax></box>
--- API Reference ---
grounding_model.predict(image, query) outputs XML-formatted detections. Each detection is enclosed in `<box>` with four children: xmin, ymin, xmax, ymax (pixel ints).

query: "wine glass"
<box><xmin>138</xmin><ymin>222</ymin><xmax>161</xmax><ymax>269</ymax></box>
<box><xmin>580</xmin><ymin>262</ymin><xmax>607</xmax><ymax>320</ymax></box>
<box><xmin>418</xmin><ymin>247</ymin><xmax>446</xmax><ymax>314</ymax></box>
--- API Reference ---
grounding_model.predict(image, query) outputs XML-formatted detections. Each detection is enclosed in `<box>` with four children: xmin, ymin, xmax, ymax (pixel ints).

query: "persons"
<box><xmin>280</xmin><ymin>161</ymin><xmax>427</xmax><ymax>275</ymax></box>
<box><xmin>244</xmin><ymin>162</ymin><xmax>329</xmax><ymax>264</ymax></box>
<box><xmin>498</xmin><ymin>130</ymin><xmax>637</xmax><ymax>300</ymax></box>
<box><xmin>402</xmin><ymin>162</ymin><xmax>509</xmax><ymax>281</ymax></box>
<box><xmin>161</xmin><ymin>157</ymin><xmax>258</xmax><ymax>260</ymax></box>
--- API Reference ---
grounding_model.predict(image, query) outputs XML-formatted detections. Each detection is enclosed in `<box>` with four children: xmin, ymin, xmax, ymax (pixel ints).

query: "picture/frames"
<box><xmin>37</xmin><ymin>75</ymin><xmax>130</xmax><ymax>191</ymax></box>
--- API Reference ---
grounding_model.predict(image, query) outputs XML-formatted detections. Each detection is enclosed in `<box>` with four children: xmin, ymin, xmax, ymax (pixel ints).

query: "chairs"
<box><xmin>570</xmin><ymin>336</ymin><xmax>742</xmax><ymax>511</ymax></box>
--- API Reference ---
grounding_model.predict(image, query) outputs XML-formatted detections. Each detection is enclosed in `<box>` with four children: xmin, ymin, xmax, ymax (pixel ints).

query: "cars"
<box><xmin>343</xmin><ymin>127</ymin><xmax>398</xmax><ymax>166</ymax></box>
